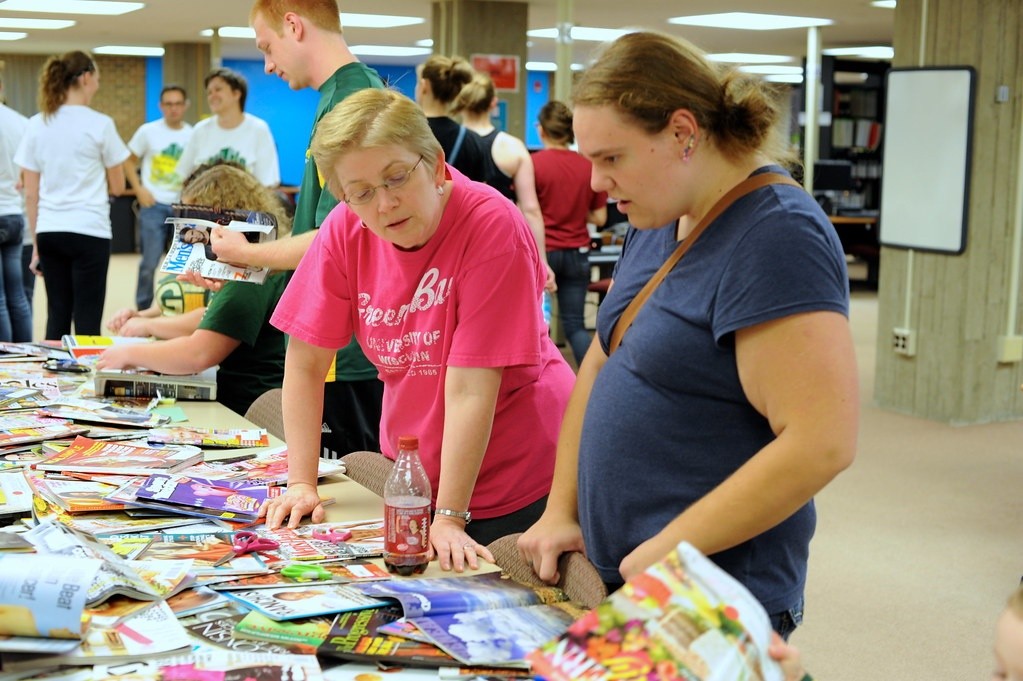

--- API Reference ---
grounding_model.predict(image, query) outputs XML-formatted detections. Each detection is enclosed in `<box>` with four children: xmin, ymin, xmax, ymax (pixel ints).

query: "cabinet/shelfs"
<box><xmin>798</xmin><ymin>59</ymin><xmax>891</xmax><ymax>213</ymax></box>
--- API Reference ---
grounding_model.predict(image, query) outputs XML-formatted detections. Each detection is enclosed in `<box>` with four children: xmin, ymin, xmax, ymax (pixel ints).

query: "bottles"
<box><xmin>383</xmin><ymin>435</ymin><xmax>433</xmax><ymax>577</ymax></box>
<box><xmin>540</xmin><ymin>287</ymin><xmax>552</xmax><ymax>338</ymax></box>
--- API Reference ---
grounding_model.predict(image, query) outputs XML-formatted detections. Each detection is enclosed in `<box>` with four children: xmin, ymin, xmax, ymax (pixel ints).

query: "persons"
<box><xmin>177</xmin><ymin>69</ymin><xmax>280</xmax><ymax>189</ymax></box>
<box><xmin>518</xmin><ymin>32</ymin><xmax>860</xmax><ymax>642</ymax></box>
<box><xmin>258</xmin><ymin>89</ymin><xmax>577</xmax><ymax>573</ymax></box>
<box><xmin>415</xmin><ymin>56</ymin><xmax>608</xmax><ymax>367</ymax></box>
<box><xmin>769</xmin><ymin>586</ymin><xmax>1023</xmax><ymax>681</ymax></box>
<box><xmin>96</xmin><ymin>161</ymin><xmax>286</xmax><ymax>418</ymax></box>
<box><xmin>150</xmin><ymin>429</ymin><xmax>234</xmax><ymax>442</ymax></box>
<box><xmin>273</xmin><ymin>586</ymin><xmax>363</xmax><ymax>608</ymax></box>
<box><xmin>179</xmin><ymin>226</ymin><xmax>260</xmax><ymax>269</ymax></box>
<box><xmin>125</xmin><ymin>87</ymin><xmax>192</xmax><ymax>311</ymax></box>
<box><xmin>14</xmin><ymin>51</ymin><xmax>131</xmax><ymax>339</ymax></box>
<box><xmin>211</xmin><ymin>1</ymin><xmax>384</xmax><ymax>460</ymax></box>
<box><xmin>0</xmin><ymin>81</ymin><xmax>36</xmax><ymax>343</ymax></box>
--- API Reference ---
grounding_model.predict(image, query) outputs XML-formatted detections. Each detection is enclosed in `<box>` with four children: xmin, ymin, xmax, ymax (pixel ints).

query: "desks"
<box><xmin>0</xmin><ymin>342</ymin><xmax>588</xmax><ymax>681</ymax></box>
<box><xmin>828</xmin><ymin>216</ymin><xmax>880</xmax><ymax>293</ymax></box>
<box><xmin>108</xmin><ymin>188</ymin><xmax>143</xmax><ymax>256</ymax></box>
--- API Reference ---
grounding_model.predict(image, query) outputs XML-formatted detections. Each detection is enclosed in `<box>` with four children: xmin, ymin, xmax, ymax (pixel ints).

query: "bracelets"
<box><xmin>435</xmin><ymin>509</ymin><xmax>471</xmax><ymax>523</ymax></box>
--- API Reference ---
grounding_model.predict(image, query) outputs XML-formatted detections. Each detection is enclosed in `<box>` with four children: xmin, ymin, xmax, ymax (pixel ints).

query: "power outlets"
<box><xmin>891</xmin><ymin>326</ymin><xmax>917</xmax><ymax>357</ymax></box>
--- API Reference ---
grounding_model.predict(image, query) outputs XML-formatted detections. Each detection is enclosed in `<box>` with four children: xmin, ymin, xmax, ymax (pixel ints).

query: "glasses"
<box><xmin>343</xmin><ymin>156</ymin><xmax>423</xmax><ymax>206</ymax></box>
<box><xmin>163</xmin><ymin>101</ymin><xmax>183</xmax><ymax>107</ymax></box>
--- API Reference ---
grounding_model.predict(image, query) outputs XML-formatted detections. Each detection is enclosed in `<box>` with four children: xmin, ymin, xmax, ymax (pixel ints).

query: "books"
<box><xmin>0</xmin><ymin>334</ymin><xmax>783</xmax><ymax>681</ymax></box>
<box><xmin>160</xmin><ymin>203</ymin><xmax>278</xmax><ymax>285</ymax></box>
<box><xmin>833</xmin><ymin>94</ymin><xmax>882</xmax><ymax>205</ymax></box>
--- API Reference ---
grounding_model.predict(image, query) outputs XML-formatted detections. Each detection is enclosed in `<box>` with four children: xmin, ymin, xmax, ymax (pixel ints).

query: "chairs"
<box><xmin>338</xmin><ymin>452</ymin><xmax>397</xmax><ymax>502</ymax></box>
<box><xmin>243</xmin><ymin>389</ymin><xmax>284</xmax><ymax>444</ymax></box>
<box><xmin>482</xmin><ymin>532</ymin><xmax>607</xmax><ymax>610</ymax></box>
